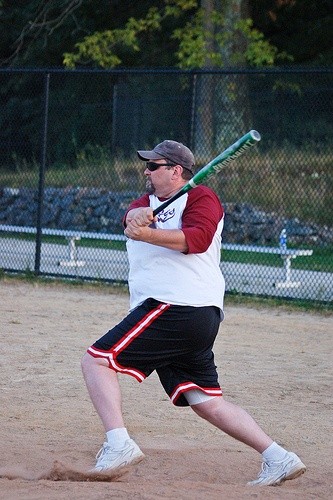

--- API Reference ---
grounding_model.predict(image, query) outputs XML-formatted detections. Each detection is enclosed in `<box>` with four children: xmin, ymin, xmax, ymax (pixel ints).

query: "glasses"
<box><xmin>145</xmin><ymin>161</ymin><xmax>175</xmax><ymax>171</ymax></box>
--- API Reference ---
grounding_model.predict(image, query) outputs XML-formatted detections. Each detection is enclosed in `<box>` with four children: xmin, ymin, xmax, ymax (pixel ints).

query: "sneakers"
<box><xmin>247</xmin><ymin>452</ymin><xmax>307</xmax><ymax>487</ymax></box>
<box><xmin>89</xmin><ymin>438</ymin><xmax>144</xmax><ymax>476</ymax></box>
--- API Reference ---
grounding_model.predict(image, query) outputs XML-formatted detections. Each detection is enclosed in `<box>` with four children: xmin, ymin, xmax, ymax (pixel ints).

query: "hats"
<box><xmin>138</xmin><ymin>140</ymin><xmax>195</xmax><ymax>176</ymax></box>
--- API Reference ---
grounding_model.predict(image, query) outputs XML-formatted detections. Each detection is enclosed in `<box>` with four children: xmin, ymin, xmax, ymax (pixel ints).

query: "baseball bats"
<box><xmin>153</xmin><ymin>130</ymin><xmax>261</xmax><ymax>216</ymax></box>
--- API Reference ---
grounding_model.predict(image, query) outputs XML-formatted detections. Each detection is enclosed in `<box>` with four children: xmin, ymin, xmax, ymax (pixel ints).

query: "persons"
<box><xmin>81</xmin><ymin>139</ymin><xmax>307</xmax><ymax>488</ymax></box>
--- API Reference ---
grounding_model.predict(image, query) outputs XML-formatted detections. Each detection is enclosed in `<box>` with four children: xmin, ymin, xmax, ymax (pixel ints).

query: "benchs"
<box><xmin>1</xmin><ymin>224</ymin><xmax>313</xmax><ymax>288</ymax></box>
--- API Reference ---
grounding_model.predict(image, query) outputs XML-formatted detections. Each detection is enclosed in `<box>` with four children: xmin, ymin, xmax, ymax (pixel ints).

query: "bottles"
<box><xmin>279</xmin><ymin>228</ymin><xmax>288</xmax><ymax>251</ymax></box>
